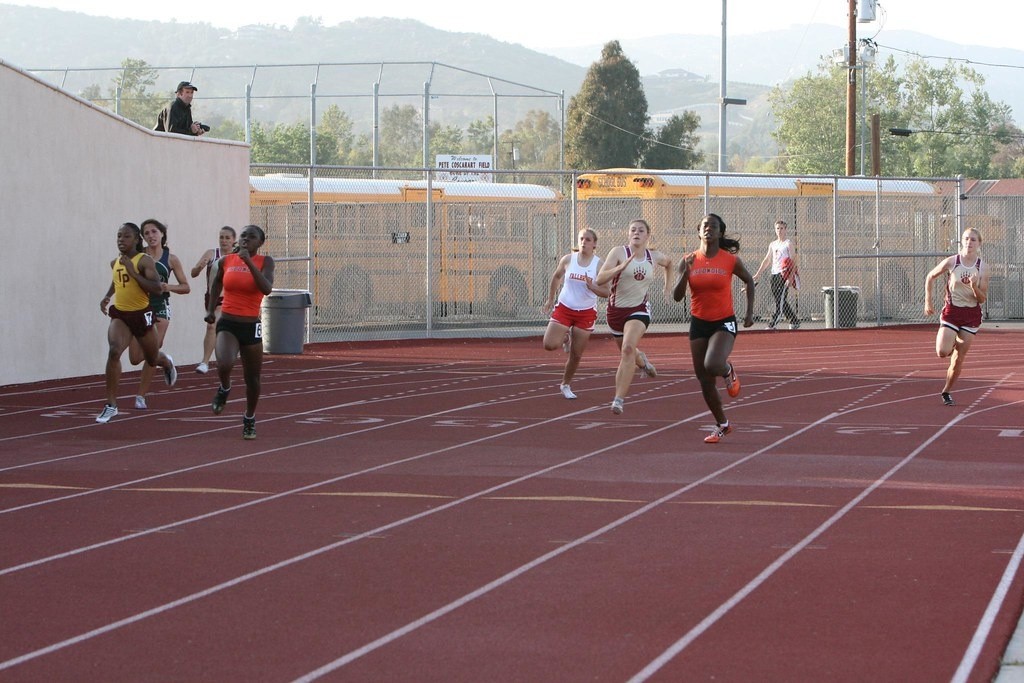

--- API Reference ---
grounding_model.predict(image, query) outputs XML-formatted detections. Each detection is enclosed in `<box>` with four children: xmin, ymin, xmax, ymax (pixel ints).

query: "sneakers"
<box><xmin>612</xmin><ymin>397</ymin><xmax>623</xmax><ymax>415</ymax></box>
<box><xmin>135</xmin><ymin>395</ymin><xmax>147</xmax><ymax>408</ymax></box>
<box><xmin>243</xmin><ymin>410</ymin><xmax>256</xmax><ymax>440</ymax></box>
<box><xmin>563</xmin><ymin>329</ymin><xmax>571</xmax><ymax>352</ymax></box>
<box><xmin>704</xmin><ymin>423</ymin><xmax>733</xmax><ymax>443</ymax></box>
<box><xmin>560</xmin><ymin>384</ymin><xmax>577</xmax><ymax>398</ymax></box>
<box><xmin>941</xmin><ymin>391</ymin><xmax>955</xmax><ymax>406</ymax></box>
<box><xmin>639</xmin><ymin>352</ymin><xmax>656</xmax><ymax>377</ymax></box>
<box><xmin>213</xmin><ymin>383</ymin><xmax>232</xmax><ymax>413</ymax></box>
<box><xmin>723</xmin><ymin>362</ymin><xmax>740</xmax><ymax>397</ymax></box>
<box><xmin>96</xmin><ymin>405</ymin><xmax>118</xmax><ymax>422</ymax></box>
<box><xmin>163</xmin><ymin>355</ymin><xmax>177</xmax><ymax>386</ymax></box>
<box><xmin>196</xmin><ymin>362</ymin><xmax>209</xmax><ymax>373</ymax></box>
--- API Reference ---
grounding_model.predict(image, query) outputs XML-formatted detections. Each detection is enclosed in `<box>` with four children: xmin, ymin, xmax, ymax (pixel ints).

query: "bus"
<box><xmin>248</xmin><ymin>174</ymin><xmax>565</xmax><ymax>321</ymax></box>
<box><xmin>570</xmin><ymin>165</ymin><xmax>943</xmax><ymax>321</ymax></box>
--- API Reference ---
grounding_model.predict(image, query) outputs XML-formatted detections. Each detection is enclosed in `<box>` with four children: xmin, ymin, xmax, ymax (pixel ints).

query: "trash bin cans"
<box><xmin>821</xmin><ymin>286</ymin><xmax>858</xmax><ymax>328</ymax></box>
<box><xmin>261</xmin><ymin>292</ymin><xmax>312</xmax><ymax>354</ymax></box>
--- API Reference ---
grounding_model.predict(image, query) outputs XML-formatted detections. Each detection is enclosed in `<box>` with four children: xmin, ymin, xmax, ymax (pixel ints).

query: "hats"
<box><xmin>175</xmin><ymin>82</ymin><xmax>197</xmax><ymax>93</ymax></box>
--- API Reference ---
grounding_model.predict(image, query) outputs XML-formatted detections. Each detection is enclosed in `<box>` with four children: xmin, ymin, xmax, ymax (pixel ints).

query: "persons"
<box><xmin>925</xmin><ymin>229</ymin><xmax>990</xmax><ymax>406</ymax></box>
<box><xmin>95</xmin><ymin>220</ymin><xmax>190</xmax><ymax>424</ymax></box>
<box><xmin>673</xmin><ymin>213</ymin><xmax>755</xmax><ymax>444</ymax></box>
<box><xmin>542</xmin><ymin>229</ymin><xmax>610</xmax><ymax>399</ymax></box>
<box><xmin>153</xmin><ymin>81</ymin><xmax>210</xmax><ymax>135</ymax></box>
<box><xmin>751</xmin><ymin>222</ymin><xmax>802</xmax><ymax>330</ymax></box>
<box><xmin>192</xmin><ymin>225</ymin><xmax>275</xmax><ymax>440</ymax></box>
<box><xmin>596</xmin><ymin>220</ymin><xmax>673</xmax><ymax>414</ymax></box>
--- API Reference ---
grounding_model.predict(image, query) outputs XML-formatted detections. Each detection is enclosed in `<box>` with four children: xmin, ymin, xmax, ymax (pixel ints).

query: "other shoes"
<box><xmin>789</xmin><ymin>323</ymin><xmax>800</xmax><ymax>329</ymax></box>
<box><xmin>765</xmin><ymin>327</ymin><xmax>776</xmax><ymax>330</ymax></box>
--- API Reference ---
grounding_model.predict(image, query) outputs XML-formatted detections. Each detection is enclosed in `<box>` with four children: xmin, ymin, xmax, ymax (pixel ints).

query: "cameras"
<box><xmin>195</xmin><ymin>122</ymin><xmax>210</xmax><ymax>132</ymax></box>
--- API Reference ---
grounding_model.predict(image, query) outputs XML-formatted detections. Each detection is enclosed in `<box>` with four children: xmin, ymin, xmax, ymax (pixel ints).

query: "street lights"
<box><xmin>718</xmin><ymin>94</ymin><xmax>747</xmax><ymax>174</ymax></box>
<box><xmin>859</xmin><ymin>45</ymin><xmax>876</xmax><ymax>177</ymax></box>
